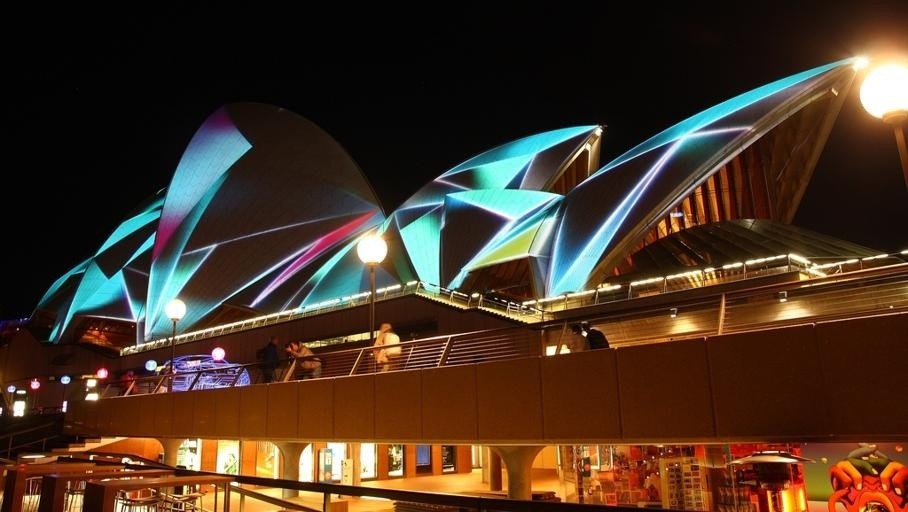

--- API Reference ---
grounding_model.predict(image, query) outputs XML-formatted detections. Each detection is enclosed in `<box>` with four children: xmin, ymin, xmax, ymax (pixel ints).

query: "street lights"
<box><xmin>357</xmin><ymin>234</ymin><xmax>387</xmax><ymax>374</ymax></box>
<box><xmin>859</xmin><ymin>64</ymin><xmax>908</xmax><ymax>182</ymax></box>
<box><xmin>164</xmin><ymin>298</ymin><xmax>187</xmax><ymax>392</ymax></box>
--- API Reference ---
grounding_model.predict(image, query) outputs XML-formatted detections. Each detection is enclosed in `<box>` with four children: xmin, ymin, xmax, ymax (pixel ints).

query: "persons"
<box><xmin>284</xmin><ymin>341</ymin><xmax>321</xmax><ymax>379</ymax></box>
<box><xmin>282</xmin><ymin>344</ymin><xmax>303</xmax><ymax>380</ymax></box>
<box><xmin>262</xmin><ymin>335</ymin><xmax>279</xmax><ymax>382</ymax></box>
<box><xmin>581</xmin><ymin>321</ymin><xmax>610</xmax><ymax>350</ymax></box>
<box><xmin>372</xmin><ymin>323</ymin><xmax>401</xmax><ymax>372</ymax></box>
